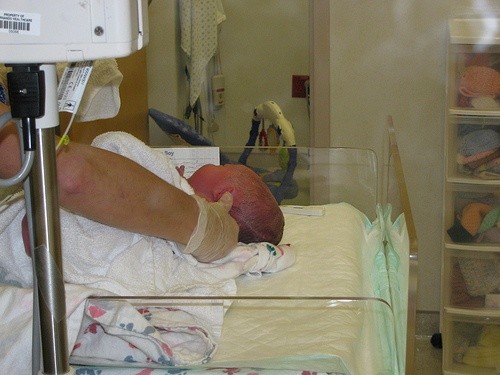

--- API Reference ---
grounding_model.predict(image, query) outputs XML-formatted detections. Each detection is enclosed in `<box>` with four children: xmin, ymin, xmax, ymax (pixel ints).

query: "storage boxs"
<box><xmin>439</xmin><ymin>17</ymin><xmax>500</xmax><ymax>375</ymax></box>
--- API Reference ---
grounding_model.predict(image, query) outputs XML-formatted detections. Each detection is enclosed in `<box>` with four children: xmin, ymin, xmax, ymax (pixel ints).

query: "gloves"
<box><xmin>180</xmin><ymin>191</ymin><xmax>239</xmax><ymax>262</ymax></box>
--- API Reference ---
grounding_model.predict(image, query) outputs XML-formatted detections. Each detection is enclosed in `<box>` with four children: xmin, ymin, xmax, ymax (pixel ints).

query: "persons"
<box><xmin>0</xmin><ymin>82</ymin><xmax>239</xmax><ymax>259</ymax></box>
<box><xmin>1</xmin><ymin>131</ymin><xmax>286</xmax><ymax>285</ymax></box>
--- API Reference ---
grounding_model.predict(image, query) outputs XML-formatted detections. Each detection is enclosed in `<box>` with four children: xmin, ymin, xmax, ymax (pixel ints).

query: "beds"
<box><xmin>0</xmin><ymin>200</ymin><xmax>373</xmax><ymax>375</ymax></box>
